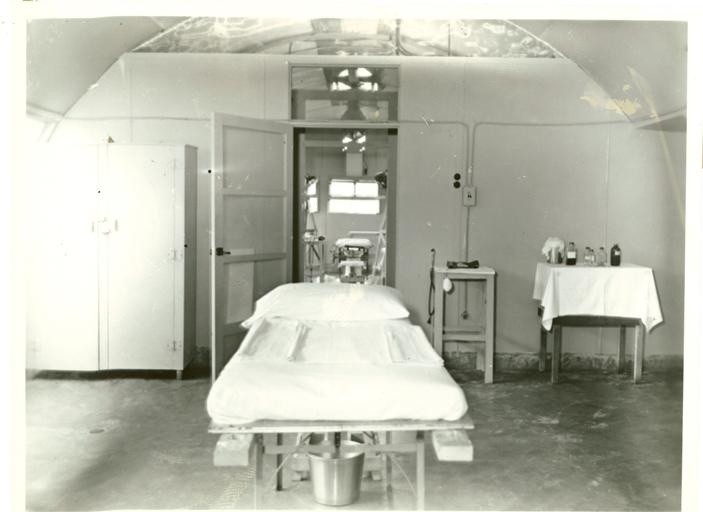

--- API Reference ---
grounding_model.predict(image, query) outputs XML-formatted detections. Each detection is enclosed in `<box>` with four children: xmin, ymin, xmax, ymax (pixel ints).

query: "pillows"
<box><xmin>242</xmin><ymin>283</ymin><xmax>410</xmax><ymax>326</ymax></box>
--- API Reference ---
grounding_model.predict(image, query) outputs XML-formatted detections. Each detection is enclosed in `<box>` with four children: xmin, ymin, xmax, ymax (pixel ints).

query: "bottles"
<box><xmin>590</xmin><ymin>249</ymin><xmax>596</xmax><ymax>264</ymax></box>
<box><xmin>596</xmin><ymin>247</ymin><xmax>607</xmax><ymax>266</ymax></box>
<box><xmin>584</xmin><ymin>246</ymin><xmax>591</xmax><ymax>264</ymax></box>
<box><xmin>566</xmin><ymin>242</ymin><xmax>577</xmax><ymax>264</ymax></box>
<box><xmin>610</xmin><ymin>245</ymin><xmax>621</xmax><ymax>266</ymax></box>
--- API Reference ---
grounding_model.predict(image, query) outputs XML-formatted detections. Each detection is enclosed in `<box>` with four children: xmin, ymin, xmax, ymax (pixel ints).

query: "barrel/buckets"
<box><xmin>304</xmin><ymin>441</ymin><xmax>364</xmax><ymax>506</ymax></box>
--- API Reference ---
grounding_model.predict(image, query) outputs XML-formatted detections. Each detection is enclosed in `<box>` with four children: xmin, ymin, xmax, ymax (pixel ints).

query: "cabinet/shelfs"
<box><xmin>26</xmin><ymin>142</ymin><xmax>198</xmax><ymax>381</ymax></box>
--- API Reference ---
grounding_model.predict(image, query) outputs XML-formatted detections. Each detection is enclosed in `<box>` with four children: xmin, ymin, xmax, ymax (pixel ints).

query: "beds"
<box><xmin>205</xmin><ymin>284</ymin><xmax>475</xmax><ymax>509</ymax></box>
<box><xmin>334</xmin><ymin>238</ymin><xmax>370</xmax><ymax>282</ymax></box>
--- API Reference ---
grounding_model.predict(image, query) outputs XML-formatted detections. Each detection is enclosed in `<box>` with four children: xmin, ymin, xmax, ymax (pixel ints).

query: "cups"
<box><xmin>547</xmin><ymin>247</ymin><xmax>562</xmax><ymax>264</ymax></box>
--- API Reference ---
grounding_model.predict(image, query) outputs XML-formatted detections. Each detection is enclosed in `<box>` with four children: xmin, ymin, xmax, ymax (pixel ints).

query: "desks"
<box><xmin>435</xmin><ymin>267</ymin><xmax>497</xmax><ymax>385</ymax></box>
<box><xmin>533</xmin><ymin>261</ymin><xmax>663</xmax><ymax>384</ymax></box>
